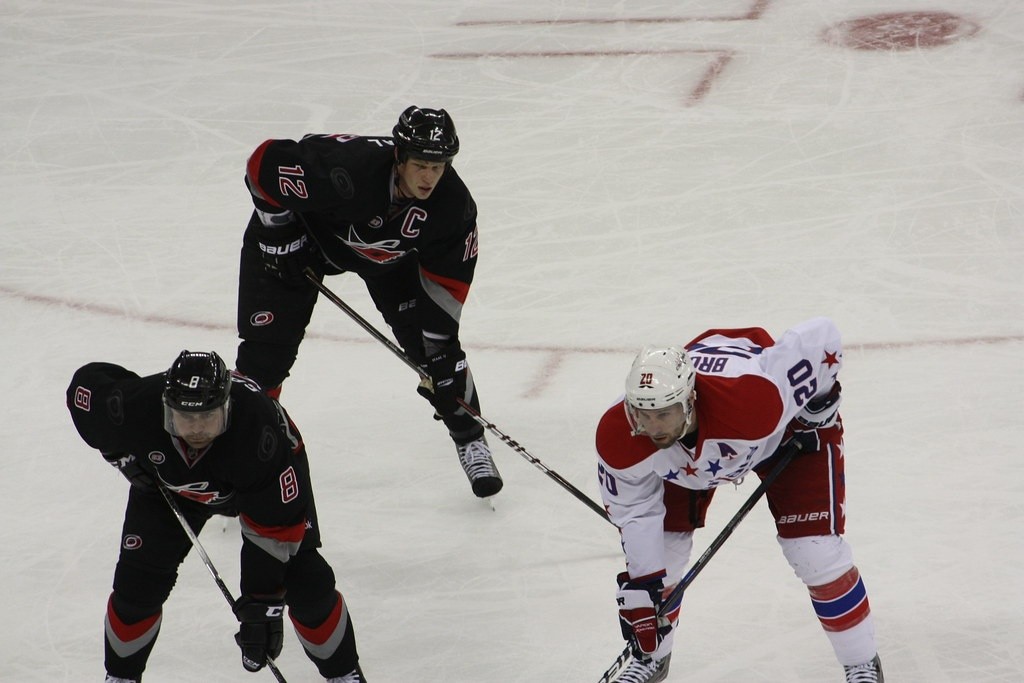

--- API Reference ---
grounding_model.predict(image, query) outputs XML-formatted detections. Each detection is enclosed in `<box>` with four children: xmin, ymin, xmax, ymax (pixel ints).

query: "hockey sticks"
<box><xmin>598</xmin><ymin>441</ymin><xmax>802</xmax><ymax>683</ymax></box>
<box><xmin>159</xmin><ymin>485</ymin><xmax>288</xmax><ymax>683</ymax></box>
<box><xmin>303</xmin><ymin>275</ymin><xmax>620</xmax><ymax>528</ymax></box>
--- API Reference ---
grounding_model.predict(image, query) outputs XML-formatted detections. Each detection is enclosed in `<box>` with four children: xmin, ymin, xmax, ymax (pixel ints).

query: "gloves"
<box><xmin>617</xmin><ymin>569</ymin><xmax>673</xmax><ymax>664</ymax></box>
<box><xmin>777</xmin><ymin>415</ymin><xmax>842</xmax><ymax>454</ymax></box>
<box><xmin>234</xmin><ymin>594</ymin><xmax>285</xmax><ymax>672</ymax></box>
<box><xmin>259</xmin><ymin>220</ymin><xmax>318</xmax><ymax>282</ymax></box>
<box><xmin>426</xmin><ymin>341</ymin><xmax>468</xmax><ymax>415</ymax></box>
<box><xmin>117</xmin><ymin>443</ymin><xmax>171</xmax><ymax>503</ymax></box>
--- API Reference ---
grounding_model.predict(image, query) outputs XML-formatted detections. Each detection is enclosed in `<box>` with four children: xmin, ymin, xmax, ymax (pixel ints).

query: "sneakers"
<box><xmin>844</xmin><ymin>652</ymin><xmax>884</xmax><ymax>683</ymax></box>
<box><xmin>598</xmin><ymin>643</ymin><xmax>672</xmax><ymax>683</ymax></box>
<box><xmin>327</xmin><ymin>664</ymin><xmax>367</xmax><ymax>683</ymax></box>
<box><xmin>456</xmin><ymin>433</ymin><xmax>503</xmax><ymax>511</ymax></box>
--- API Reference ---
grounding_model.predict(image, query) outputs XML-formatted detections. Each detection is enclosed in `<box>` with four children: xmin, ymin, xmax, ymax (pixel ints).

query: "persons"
<box><xmin>65</xmin><ymin>351</ymin><xmax>368</xmax><ymax>683</ymax></box>
<box><xmin>595</xmin><ymin>318</ymin><xmax>885</xmax><ymax>683</ymax></box>
<box><xmin>234</xmin><ymin>106</ymin><xmax>504</xmax><ymax>510</ymax></box>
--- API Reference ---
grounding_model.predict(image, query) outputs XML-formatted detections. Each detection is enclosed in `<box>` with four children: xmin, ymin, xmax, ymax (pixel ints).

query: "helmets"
<box><xmin>162</xmin><ymin>349</ymin><xmax>231</xmax><ymax>441</ymax></box>
<box><xmin>625</xmin><ymin>344</ymin><xmax>696</xmax><ymax>440</ymax></box>
<box><xmin>392</xmin><ymin>105</ymin><xmax>459</xmax><ymax>182</ymax></box>
